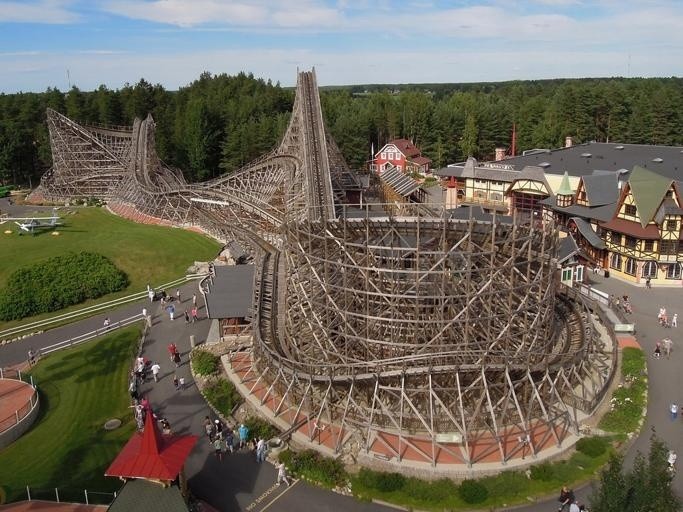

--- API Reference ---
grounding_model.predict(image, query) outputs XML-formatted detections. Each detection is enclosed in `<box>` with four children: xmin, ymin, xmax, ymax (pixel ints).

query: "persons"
<box><xmin>556</xmin><ymin>485</ymin><xmax>571</xmax><ymax>511</ymax></box>
<box><xmin>667</xmin><ymin>465</ymin><xmax>676</xmax><ymax>476</ymax></box>
<box><xmin>201</xmin><ymin>415</ymin><xmax>265</xmax><ymax>462</ymax></box>
<box><xmin>274</xmin><ymin>459</ymin><xmax>290</xmax><ymax>486</ymax></box>
<box><xmin>670</xmin><ymin>402</ymin><xmax>678</xmax><ymax>419</ymax></box>
<box><xmin>668</xmin><ymin>449</ymin><xmax>676</xmax><ymax>472</ymax></box>
<box><xmin>28</xmin><ymin>348</ymin><xmax>35</xmax><ymax>364</ymax></box>
<box><xmin>610</xmin><ymin>292</ymin><xmax>633</xmax><ymax>315</ymax></box>
<box><xmin>569</xmin><ymin>500</ymin><xmax>580</xmax><ymax>512</ymax></box>
<box><xmin>579</xmin><ymin>505</ymin><xmax>589</xmax><ymax>512</ymax></box>
<box><xmin>653</xmin><ymin>305</ymin><xmax>677</xmax><ymax>360</ymax></box>
<box><xmin>644</xmin><ymin>273</ymin><xmax>651</xmax><ymax>289</ymax></box>
<box><xmin>142</xmin><ymin>283</ymin><xmax>198</xmax><ymax>329</ymax></box>
<box><xmin>103</xmin><ymin>317</ymin><xmax>110</xmax><ymax>330</ymax></box>
<box><xmin>128</xmin><ymin>342</ymin><xmax>185</xmax><ymax>433</ymax></box>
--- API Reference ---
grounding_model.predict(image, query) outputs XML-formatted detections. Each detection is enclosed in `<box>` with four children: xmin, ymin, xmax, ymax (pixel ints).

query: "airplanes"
<box><xmin>0</xmin><ymin>207</ymin><xmax>63</xmax><ymax>236</ymax></box>
